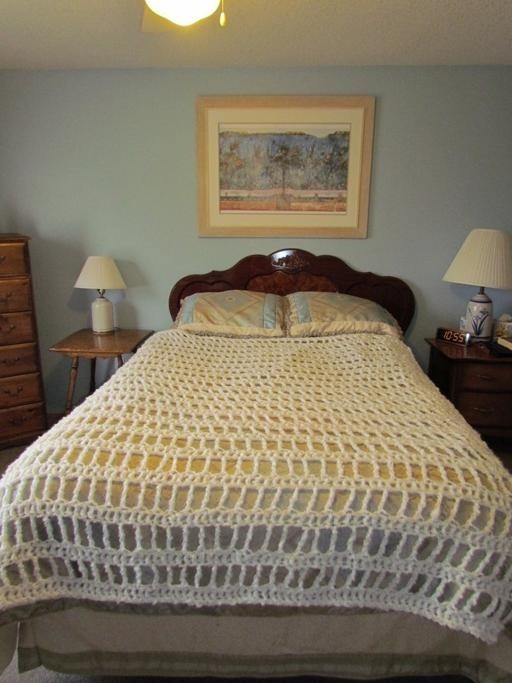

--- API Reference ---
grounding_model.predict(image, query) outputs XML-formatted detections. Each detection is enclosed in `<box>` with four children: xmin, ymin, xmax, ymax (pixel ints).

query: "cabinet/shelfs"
<box><xmin>0</xmin><ymin>230</ymin><xmax>49</xmax><ymax>451</ymax></box>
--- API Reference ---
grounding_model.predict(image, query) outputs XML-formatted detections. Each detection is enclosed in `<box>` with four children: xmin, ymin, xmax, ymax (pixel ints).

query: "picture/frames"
<box><xmin>192</xmin><ymin>92</ymin><xmax>377</xmax><ymax>240</ymax></box>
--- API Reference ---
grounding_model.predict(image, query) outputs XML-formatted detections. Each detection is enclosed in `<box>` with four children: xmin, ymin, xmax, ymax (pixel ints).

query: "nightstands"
<box><xmin>424</xmin><ymin>335</ymin><xmax>511</xmax><ymax>454</ymax></box>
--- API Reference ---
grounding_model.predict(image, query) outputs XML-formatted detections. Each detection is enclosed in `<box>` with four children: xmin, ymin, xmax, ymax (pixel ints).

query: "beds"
<box><xmin>0</xmin><ymin>248</ymin><xmax>510</xmax><ymax>683</ymax></box>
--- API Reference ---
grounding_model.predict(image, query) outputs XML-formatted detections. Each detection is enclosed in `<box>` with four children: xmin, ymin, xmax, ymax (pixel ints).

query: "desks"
<box><xmin>47</xmin><ymin>326</ymin><xmax>152</xmax><ymax>419</ymax></box>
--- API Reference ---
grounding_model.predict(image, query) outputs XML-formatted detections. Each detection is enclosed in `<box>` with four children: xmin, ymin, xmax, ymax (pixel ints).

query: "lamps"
<box><xmin>73</xmin><ymin>256</ymin><xmax>126</xmax><ymax>334</ymax></box>
<box><xmin>438</xmin><ymin>226</ymin><xmax>512</xmax><ymax>345</ymax></box>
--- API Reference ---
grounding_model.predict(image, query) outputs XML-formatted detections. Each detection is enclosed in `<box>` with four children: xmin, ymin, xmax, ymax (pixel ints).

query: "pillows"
<box><xmin>175</xmin><ymin>290</ymin><xmax>401</xmax><ymax>339</ymax></box>
